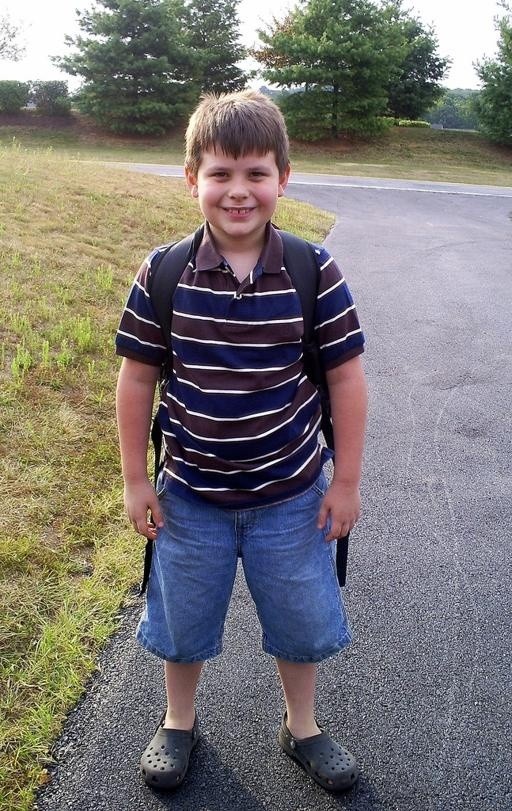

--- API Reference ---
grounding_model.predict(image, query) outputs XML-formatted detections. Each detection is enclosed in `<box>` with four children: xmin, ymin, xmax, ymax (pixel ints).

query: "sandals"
<box><xmin>278</xmin><ymin>709</ymin><xmax>359</xmax><ymax>791</ymax></box>
<box><xmin>140</xmin><ymin>706</ymin><xmax>202</xmax><ymax>790</ymax></box>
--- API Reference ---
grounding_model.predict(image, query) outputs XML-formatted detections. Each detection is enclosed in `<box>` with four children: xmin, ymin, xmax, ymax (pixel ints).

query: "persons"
<box><xmin>114</xmin><ymin>90</ymin><xmax>365</xmax><ymax>793</ymax></box>
